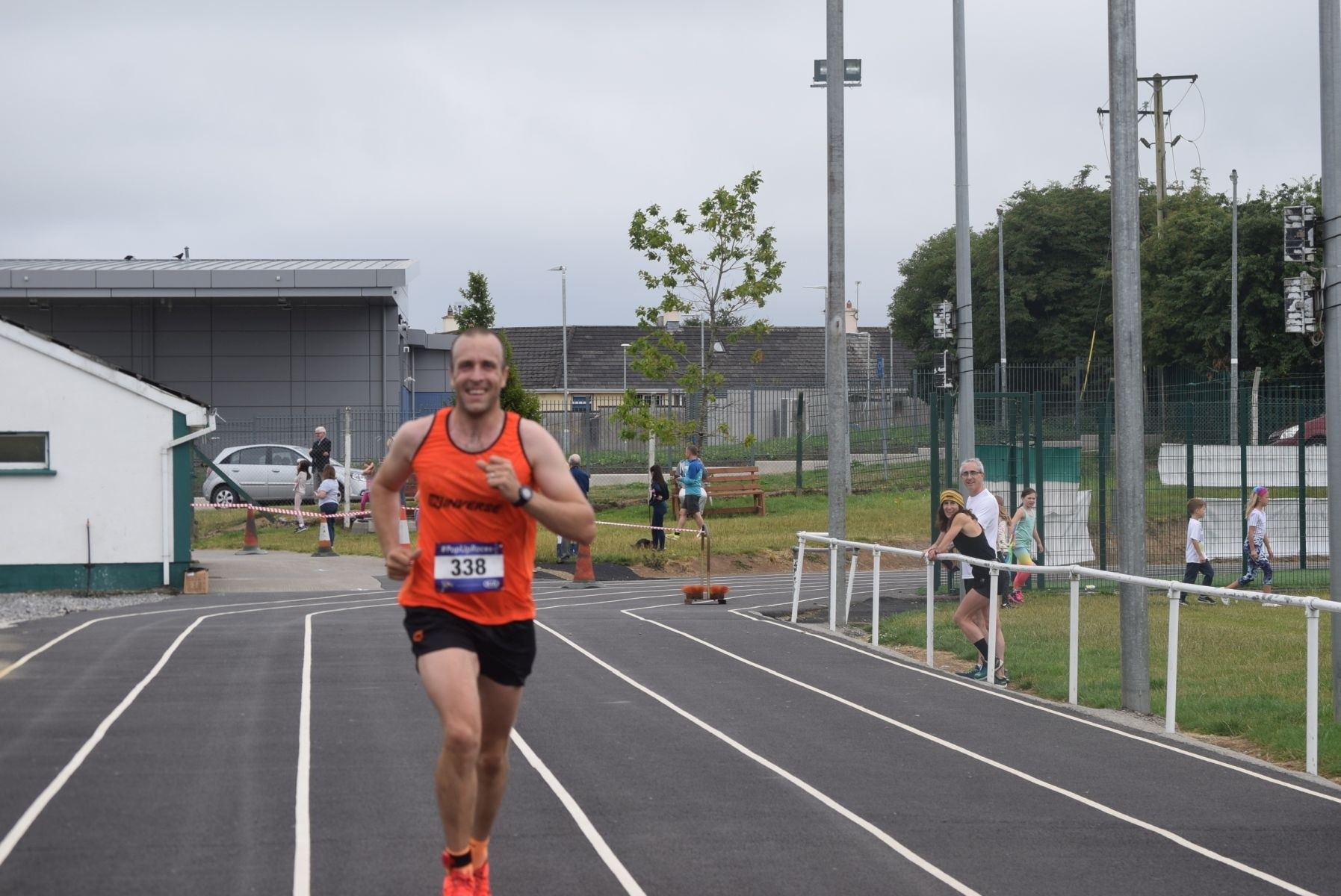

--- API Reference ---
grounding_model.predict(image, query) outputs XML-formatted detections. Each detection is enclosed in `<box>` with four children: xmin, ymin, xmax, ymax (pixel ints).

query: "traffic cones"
<box><xmin>398</xmin><ymin>504</ymin><xmax>411</xmax><ymax>550</ymax></box>
<box><xmin>561</xmin><ymin>542</ymin><xmax>603</xmax><ymax>590</ymax></box>
<box><xmin>235</xmin><ymin>504</ymin><xmax>268</xmax><ymax>554</ymax></box>
<box><xmin>310</xmin><ymin>513</ymin><xmax>340</xmax><ymax>557</ymax></box>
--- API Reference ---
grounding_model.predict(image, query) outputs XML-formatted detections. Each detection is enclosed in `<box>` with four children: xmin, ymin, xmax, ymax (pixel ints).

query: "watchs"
<box><xmin>511</xmin><ymin>484</ymin><xmax>532</xmax><ymax>508</ymax></box>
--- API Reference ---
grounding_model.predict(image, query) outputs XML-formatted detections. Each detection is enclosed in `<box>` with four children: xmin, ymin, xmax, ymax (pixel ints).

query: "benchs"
<box><xmin>404</xmin><ymin>474</ymin><xmax>417</xmax><ymax>501</ymax></box>
<box><xmin>669</xmin><ymin>466</ymin><xmax>766</xmax><ymax>521</ymax></box>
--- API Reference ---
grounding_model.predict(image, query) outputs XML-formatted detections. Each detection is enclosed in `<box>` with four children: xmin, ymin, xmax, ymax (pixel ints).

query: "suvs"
<box><xmin>202</xmin><ymin>443</ymin><xmax>370</xmax><ymax>510</ymax></box>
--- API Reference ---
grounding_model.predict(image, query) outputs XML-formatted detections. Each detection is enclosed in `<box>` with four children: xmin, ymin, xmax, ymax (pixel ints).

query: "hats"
<box><xmin>939</xmin><ymin>489</ymin><xmax>964</xmax><ymax>509</ymax></box>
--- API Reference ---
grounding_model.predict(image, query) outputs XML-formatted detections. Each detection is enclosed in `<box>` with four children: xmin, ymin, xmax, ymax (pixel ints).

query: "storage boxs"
<box><xmin>184</xmin><ymin>571</ymin><xmax>209</xmax><ymax>595</ymax></box>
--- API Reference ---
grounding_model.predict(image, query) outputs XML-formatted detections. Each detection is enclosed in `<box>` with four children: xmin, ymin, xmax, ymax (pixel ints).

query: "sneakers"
<box><xmin>694</xmin><ymin>536</ymin><xmax>707</xmax><ymax>541</ymax></box>
<box><xmin>665</xmin><ymin>532</ymin><xmax>680</xmax><ymax>541</ymax></box>
<box><xmin>1199</xmin><ymin>597</ymin><xmax>1216</xmax><ymax>604</ymax></box>
<box><xmin>474</xmin><ymin>858</ymin><xmax>488</xmax><ymax>896</ymax></box>
<box><xmin>1179</xmin><ymin>600</ymin><xmax>1189</xmax><ymax>606</ymax></box>
<box><xmin>1009</xmin><ymin>590</ymin><xmax>1024</xmax><ymax>604</ymax></box>
<box><xmin>975</xmin><ymin>655</ymin><xmax>1003</xmax><ymax>679</ymax></box>
<box><xmin>955</xmin><ymin>665</ymin><xmax>977</xmax><ymax>678</ymax></box>
<box><xmin>441</xmin><ymin>846</ymin><xmax>474</xmax><ymax>896</ymax></box>
<box><xmin>994</xmin><ymin>676</ymin><xmax>1007</xmax><ymax>688</ymax></box>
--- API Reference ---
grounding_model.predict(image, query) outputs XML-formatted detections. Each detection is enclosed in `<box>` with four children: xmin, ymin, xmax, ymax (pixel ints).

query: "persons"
<box><xmin>308</xmin><ymin>427</ymin><xmax>331</xmax><ymax>505</ymax></box>
<box><xmin>924</xmin><ymin>457</ymin><xmax>1044</xmax><ymax>689</ymax></box>
<box><xmin>675</xmin><ymin>458</ymin><xmax>707</xmax><ymax>516</ymax></box>
<box><xmin>664</xmin><ymin>445</ymin><xmax>708</xmax><ymax>541</ymax></box>
<box><xmin>561</xmin><ymin>454</ymin><xmax>589</xmax><ymax>560</ymax></box>
<box><xmin>315</xmin><ymin>465</ymin><xmax>340</xmax><ymax>548</ymax></box>
<box><xmin>1219</xmin><ymin>486</ymin><xmax>1281</xmax><ymax>607</ymax></box>
<box><xmin>649</xmin><ymin>465</ymin><xmax>669</xmax><ymax>551</ymax></box>
<box><xmin>293</xmin><ymin>460</ymin><xmax>311</xmax><ymax>532</ymax></box>
<box><xmin>357</xmin><ymin>329</ymin><xmax>596</xmax><ymax>896</ymax></box>
<box><xmin>1178</xmin><ymin>498</ymin><xmax>1217</xmax><ymax>605</ymax></box>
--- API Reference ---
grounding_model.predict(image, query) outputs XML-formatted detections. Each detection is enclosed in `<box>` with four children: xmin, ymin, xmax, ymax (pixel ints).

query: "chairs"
<box><xmin>274</xmin><ymin>455</ymin><xmax>290</xmax><ymax>465</ymax></box>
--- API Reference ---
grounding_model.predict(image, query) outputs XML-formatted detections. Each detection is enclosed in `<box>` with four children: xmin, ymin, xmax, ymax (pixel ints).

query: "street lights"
<box><xmin>1229</xmin><ymin>168</ymin><xmax>1238</xmax><ymax>445</ymax></box>
<box><xmin>681</xmin><ymin>311</ymin><xmax>704</xmax><ymax>389</ymax></box>
<box><xmin>546</xmin><ymin>266</ymin><xmax>570</xmax><ymax>457</ymax></box>
<box><xmin>859</xmin><ymin>331</ymin><xmax>871</xmax><ymax>432</ymax></box>
<box><xmin>621</xmin><ymin>343</ymin><xmax>632</xmax><ymax>390</ymax></box>
<box><xmin>995</xmin><ymin>204</ymin><xmax>1008</xmax><ymax>431</ymax></box>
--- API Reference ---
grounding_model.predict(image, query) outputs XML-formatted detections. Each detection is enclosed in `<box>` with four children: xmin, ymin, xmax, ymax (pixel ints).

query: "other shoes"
<box><xmin>1001</xmin><ymin>603</ymin><xmax>1016</xmax><ymax>609</ymax></box>
<box><xmin>1219</xmin><ymin>587</ymin><xmax>1230</xmax><ymax>605</ymax></box>
<box><xmin>1262</xmin><ymin>603</ymin><xmax>1281</xmax><ymax>607</ymax></box>
<box><xmin>294</xmin><ymin>526</ymin><xmax>306</xmax><ymax>533</ymax></box>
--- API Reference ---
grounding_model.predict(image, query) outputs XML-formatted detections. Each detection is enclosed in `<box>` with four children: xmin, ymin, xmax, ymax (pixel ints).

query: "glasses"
<box><xmin>960</xmin><ymin>471</ymin><xmax>982</xmax><ymax>478</ymax></box>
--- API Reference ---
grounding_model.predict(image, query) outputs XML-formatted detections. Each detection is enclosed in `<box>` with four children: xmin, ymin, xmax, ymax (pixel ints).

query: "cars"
<box><xmin>1266</xmin><ymin>414</ymin><xmax>1327</xmax><ymax>446</ymax></box>
